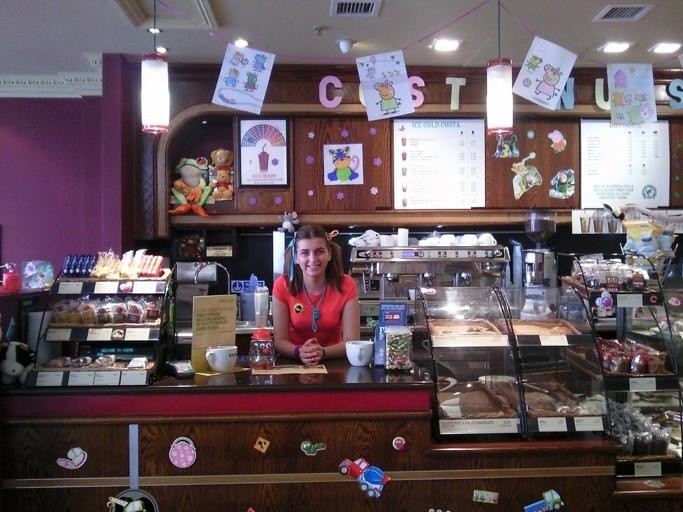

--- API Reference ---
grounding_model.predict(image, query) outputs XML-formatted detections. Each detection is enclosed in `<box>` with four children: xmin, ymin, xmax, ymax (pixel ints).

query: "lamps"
<box><xmin>138</xmin><ymin>2</ymin><xmax>171</xmax><ymax>132</ymax></box>
<box><xmin>484</xmin><ymin>0</ymin><xmax>514</xmax><ymax>137</ymax></box>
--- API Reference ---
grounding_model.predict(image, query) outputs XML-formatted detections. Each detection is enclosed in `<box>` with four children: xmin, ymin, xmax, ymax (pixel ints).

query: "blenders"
<box><xmin>521</xmin><ymin>207</ymin><xmax>559</xmax><ymax>321</ymax></box>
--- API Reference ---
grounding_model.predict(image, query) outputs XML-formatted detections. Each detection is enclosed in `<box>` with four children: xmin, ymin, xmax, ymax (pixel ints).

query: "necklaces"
<box><xmin>303</xmin><ymin>282</ymin><xmax>328</xmax><ymax>333</ymax></box>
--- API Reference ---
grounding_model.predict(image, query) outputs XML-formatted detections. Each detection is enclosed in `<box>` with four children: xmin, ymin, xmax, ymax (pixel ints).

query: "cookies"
<box><xmin>442</xmin><ymin>328</ymin><xmax>453</xmax><ymax>332</ymax></box>
<box><xmin>467</xmin><ymin>325</ymin><xmax>482</xmax><ymax>331</ymax></box>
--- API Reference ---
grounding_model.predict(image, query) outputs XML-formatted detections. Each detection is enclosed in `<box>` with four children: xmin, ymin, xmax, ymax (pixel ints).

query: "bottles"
<box><xmin>241</xmin><ymin>275</ymin><xmax>269</xmax><ymax>329</ymax></box>
<box><xmin>248</xmin><ymin>331</ymin><xmax>275</xmax><ymax>370</ymax></box>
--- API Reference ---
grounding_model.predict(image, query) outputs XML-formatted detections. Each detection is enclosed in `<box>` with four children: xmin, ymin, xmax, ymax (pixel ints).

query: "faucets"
<box><xmin>192</xmin><ymin>261</ymin><xmax>231</xmax><ymax>295</ymax></box>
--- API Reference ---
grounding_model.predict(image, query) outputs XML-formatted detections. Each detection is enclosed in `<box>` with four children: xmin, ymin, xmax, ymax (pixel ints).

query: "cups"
<box><xmin>580</xmin><ymin>216</ymin><xmax>627</xmax><ymax>233</ymax></box>
<box><xmin>345</xmin><ymin>340</ymin><xmax>374</xmax><ymax>366</ymax></box>
<box><xmin>258</xmin><ymin>152</ymin><xmax>269</xmax><ymax>170</ymax></box>
<box><xmin>2</xmin><ymin>273</ymin><xmax>19</xmax><ymax>294</ymax></box>
<box><xmin>366</xmin><ymin>227</ymin><xmax>498</xmax><ymax>259</ymax></box>
<box><xmin>205</xmin><ymin>345</ymin><xmax>238</xmax><ymax>371</ymax></box>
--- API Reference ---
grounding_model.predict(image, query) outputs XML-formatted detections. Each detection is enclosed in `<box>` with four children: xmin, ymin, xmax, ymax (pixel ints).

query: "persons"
<box><xmin>272</xmin><ymin>224</ymin><xmax>360</xmax><ymax>366</ymax></box>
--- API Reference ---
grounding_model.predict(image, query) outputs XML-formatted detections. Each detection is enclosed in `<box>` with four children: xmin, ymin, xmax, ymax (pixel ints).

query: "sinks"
<box><xmin>236</xmin><ymin>320</ymin><xmax>249</xmax><ymax>329</ymax></box>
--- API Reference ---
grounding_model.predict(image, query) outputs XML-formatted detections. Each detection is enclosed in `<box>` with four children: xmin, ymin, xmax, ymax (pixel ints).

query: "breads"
<box><xmin>603</xmin><ymin>397</ymin><xmax>668</xmax><ymax>457</ymax></box>
<box><xmin>585</xmin><ymin>338</ymin><xmax>665</xmax><ymax>374</ymax></box>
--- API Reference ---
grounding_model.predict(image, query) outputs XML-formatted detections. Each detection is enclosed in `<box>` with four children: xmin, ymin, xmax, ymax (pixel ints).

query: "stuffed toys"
<box><xmin>169</xmin><ymin>149</ymin><xmax>234</xmax><ymax>216</ymax></box>
<box><xmin>0</xmin><ymin>341</ymin><xmax>39</xmax><ymax>384</ymax></box>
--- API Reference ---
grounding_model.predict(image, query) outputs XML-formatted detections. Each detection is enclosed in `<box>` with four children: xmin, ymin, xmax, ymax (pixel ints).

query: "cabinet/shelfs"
<box><xmin>0</xmin><ymin>390</ymin><xmax>683</xmax><ymax>512</ymax></box>
